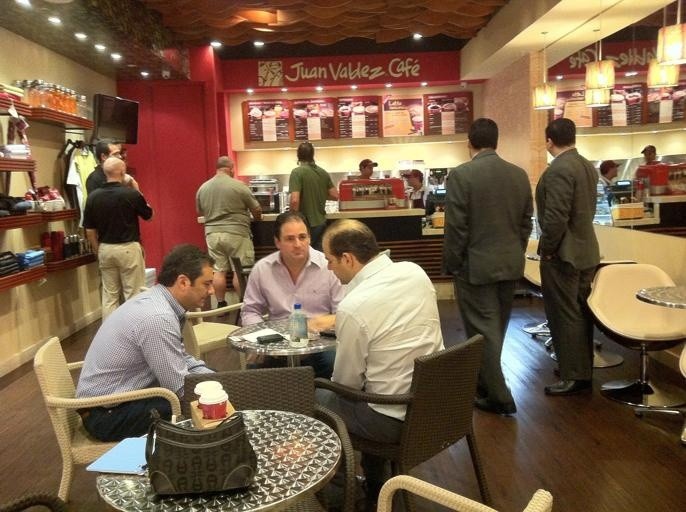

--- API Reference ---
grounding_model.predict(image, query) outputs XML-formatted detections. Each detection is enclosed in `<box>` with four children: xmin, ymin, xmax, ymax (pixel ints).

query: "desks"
<box><xmin>635</xmin><ymin>285</ymin><xmax>686</xmax><ymax>309</ymax></box>
<box><xmin>226</xmin><ymin>314</ymin><xmax>338</xmax><ymax>367</ymax></box>
<box><xmin>96</xmin><ymin>409</ymin><xmax>343</xmax><ymax>512</ymax></box>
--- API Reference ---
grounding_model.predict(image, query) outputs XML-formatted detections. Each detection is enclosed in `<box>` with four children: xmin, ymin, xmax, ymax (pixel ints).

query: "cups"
<box><xmin>388</xmin><ymin>197</ymin><xmax>414</xmax><ymax>209</ymax></box>
<box><xmin>193</xmin><ymin>380</ymin><xmax>224</xmax><ymax>405</ymax></box>
<box><xmin>304</xmin><ymin>326</ymin><xmax>321</xmax><ymax>340</ymax></box>
<box><xmin>338</xmin><ymin>106</ymin><xmax>351</xmax><ymax>118</ymax></box>
<box><xmin>197</xmin><ymin>390</ymin><xmax>229</xmax><ymax>421</ymax></box>
<box><xmin>412</xmin><ymin>117</ymin><xmax>423</xmax><ymax>131</ymax></box>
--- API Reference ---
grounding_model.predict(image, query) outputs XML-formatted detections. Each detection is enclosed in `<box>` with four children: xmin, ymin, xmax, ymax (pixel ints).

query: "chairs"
<box><xmin>550</xmin><ymin>259</ymin><xmax>638</xmax><ymax>368</ymax></box>
<box><xmin>313</xmin><ymin>332</ymin><xmax>492</xmax><ymax>510</ymax></box>
<box><xmin>33</xmin><ymin>336</ymin><xmax>181</xmax><ymax>503</ymax></box>
<box><xmin>586</xmin><ymin>263</ymin><xmax>686</xmax><ymax>408</ymax></box>
<box><xmin>0</xmin><ymin>491</ymin><xmax>71</xmax><ymax>512</ymax></box>
<box><xmin>184</xmin><ymin>365</ymin><xmax>357</xmax><ymax>512</ymax></box>
<box><xmin>377</xmin><ymin>475</ymin><xmax>556</xmax><ymax>512</ymax></box>
<box><xmin>231</xmin><ymin>255</ymin><xmax>254</xmax><ymax>327</ymax></box>
<box><xmin>523</xmin><ymin>238</ymin><xmax>551</xmax><ymax>341</ymax></box>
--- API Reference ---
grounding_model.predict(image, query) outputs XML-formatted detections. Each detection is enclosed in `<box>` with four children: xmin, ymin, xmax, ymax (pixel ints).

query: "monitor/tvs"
<box><xmin>93</xmin><ymin>93</ymin><xmax>138</xmax><ymax>145</ymax></box>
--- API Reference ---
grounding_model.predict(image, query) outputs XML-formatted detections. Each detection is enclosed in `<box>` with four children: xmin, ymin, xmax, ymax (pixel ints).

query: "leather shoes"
<box><xmin>551</xmin><ymin>365</ymin><xmax>563</xmax><ymax>377</ymax></box>
<box><xmin>474</xmin><ymin>393</ymin><xmax>517</xmax><ymax>415</ymax></box>
<box><xmin>544</xmin><ymin>377</ymin><xmax>594</xmax><ymax>397</ymax></box>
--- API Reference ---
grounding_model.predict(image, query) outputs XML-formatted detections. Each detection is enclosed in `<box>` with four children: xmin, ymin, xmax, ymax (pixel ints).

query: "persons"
<box><xmin>641</xmin><ymin>145</ymin><xmax>656</xmax><ymax>164</ymax></box>
<box><xmin>534</xmin><ymin>117</ymin><xmax>601</xmax><ymax>397</ymax></box>
<box><xmin>76</xmin><ymin>242</ymin><xmax>219</xmax><ymax>443</ymax></box>
<box><xmin>442</xmin><ymin>117</ymin><xmax>534</xmax><ymax>412</ymax></box>
<box><xmin>195</xmin><ymin>156</ymin><xmax>264</xmax><ymax>315</ymax></box>
<box><xmin>401</xmin><ymin>168</ymin><xmax>434</xmax><ymax>209</ymax></box>
<box><xmin>312</xmin><ymin>217</ymin><xmax>449</xmax><ymax>512</ymax></box>
<box><xmin>81</xmin><ymin>139</ymin><xmax>155</xmax><ymax>324</ymax></box>
<box><xmin>597</xmin><ymin>159</ymin><xmax>621</xmax><ymax>187</ymax></box>
<box><xmin>358</xmin><ymin>159</ymin><xmax>378</xmax><ymax>179</ymax></box>
<box><xmin>288</xmin><ymin>142</ymin><xmax>340</xmax><ymax>251</ymax></box>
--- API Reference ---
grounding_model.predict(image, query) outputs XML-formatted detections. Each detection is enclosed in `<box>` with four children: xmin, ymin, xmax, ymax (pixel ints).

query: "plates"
<box><xmin>365</xmin><ymin>105</ymin><xmax>379</xmax><ymax>114</ymax></box>
<box><xmin>352</xmin><ymin>106</ymin><xmax>364</xmax><ymax>114</ymax></box>
<box><xmin>611</xmin><ymin>93</ymin><xmax>624</xmax><ymax>103</ymax></box>
<box><xmin>442</xmin><ymin>103</ymin><xmax>456</xmax><ymax>111</ymax></box>
<box><xmin>428</xmin><ymin>104</ymin><xmax>441</xmax><ymax>111</ymax></box>
<box><xmin>554</xmin><ymin>108</ymin><xmax>563</xmax><ymax>115</ymax></box>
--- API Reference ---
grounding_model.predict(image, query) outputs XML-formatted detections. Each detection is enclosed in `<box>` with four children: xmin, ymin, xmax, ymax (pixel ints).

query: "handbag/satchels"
<box><xmin>144</xmin><ymin>408</ymin><xmax>259</xmax><ymax>499</ymax></box>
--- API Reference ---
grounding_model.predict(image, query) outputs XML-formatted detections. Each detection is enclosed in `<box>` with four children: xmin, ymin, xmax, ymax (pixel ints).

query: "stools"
<box><xmin>184</xmin><ymin>301</ymin><xmax>245</xmax><ymax>371</ymax></box>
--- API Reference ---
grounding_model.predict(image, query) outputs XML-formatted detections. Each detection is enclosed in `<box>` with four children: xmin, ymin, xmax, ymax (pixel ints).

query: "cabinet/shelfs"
<box><xmin>0</xmin><ymin>99</ymin><xmax>96</xmax><ymax>291</ymax></box>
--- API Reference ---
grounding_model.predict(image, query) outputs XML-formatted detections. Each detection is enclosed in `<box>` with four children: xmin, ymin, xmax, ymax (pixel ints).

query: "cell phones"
<box><xmin>320</xmin><ymin>329</ymin><xmax>335</xmax><ymax>337</ymax></box>
<box><xmin>256</xmin><ymin>334</ymin><xmax>284</xmax><ymax>343</ymax></box>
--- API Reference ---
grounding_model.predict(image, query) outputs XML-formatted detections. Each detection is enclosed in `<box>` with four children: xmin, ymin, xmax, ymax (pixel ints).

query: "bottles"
<box><xmin>40</xmin><ymin>231</ymin><xmax>84</xmax><ymax>261</ymax></box>
<box><xmin>11</xmin><ymin>79</ymin><xmax>88</xmax><ymax>120</ymax></box>
<box><xmin>287</xmin><ymin>303</ymin><xmax>309</xmax><ymax>349</ymax></box>
<box><xmin>633</xmin><ymin>179</ymin><xmax>650</xmax><ymax>198</ymax></box>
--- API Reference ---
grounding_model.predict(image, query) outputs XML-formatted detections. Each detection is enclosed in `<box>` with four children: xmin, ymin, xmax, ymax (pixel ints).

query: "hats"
<box><xmin>359</xmin><ymin>159</ymin><xmax>378</xmax><ymax>172</ymax></box>
<box><xmin>401</xmin><ymin>169</ymin><xmax>424</xmax><ymax>178</ymax></box>
<box><xmin>641</xmin><ymin>144</ymin><xmax>656</xmax><ymax>155</ymax></box>
<box><xmin>600</xmin><ymin>160</ymin><xmax>621</xmax><ymax>171</ymax></box>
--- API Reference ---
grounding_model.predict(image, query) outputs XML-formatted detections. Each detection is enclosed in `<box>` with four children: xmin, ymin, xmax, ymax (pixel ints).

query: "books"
<box><xmin>85</xmin><ymin>435</ymin><xmax>157</xmax><ymax>475</ymax></box>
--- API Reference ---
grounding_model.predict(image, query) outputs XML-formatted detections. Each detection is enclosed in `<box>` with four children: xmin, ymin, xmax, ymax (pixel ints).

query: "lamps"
<box><xmin>657</xmin><ymin>0</ymin><xmax>686</xmax><ymax>65</ymax></box>
<box><xmin>585</xmin><ymin>32</ymin><xmax>616</xmax><ymax>89</ymax></box>
<box><xmin>646</xmin><ymin>7</ymin><xmax>680</xmax><ymax>88</ymax></box>
<box><xmin>533</xmin><ymin>31</ymin><xmax>556</xmax><ymax>111</ymax></box>
<box><xmin>585</xmin><ymin>29</ymin><xmax>610</xmax><ymax>107</ymax></box>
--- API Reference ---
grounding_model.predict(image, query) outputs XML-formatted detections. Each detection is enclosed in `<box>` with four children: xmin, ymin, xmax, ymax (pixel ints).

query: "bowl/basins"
<box><xmin>625</xmin><ymin>92</ymin><xmax>642</xmax><ymax>105</ymax></box>
